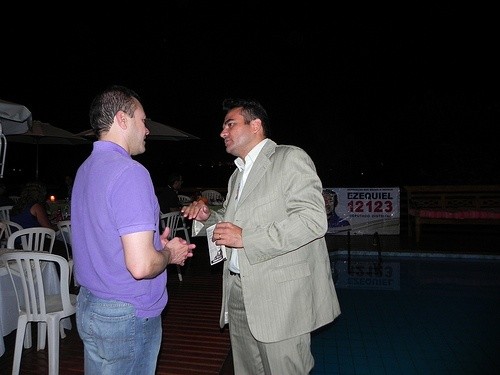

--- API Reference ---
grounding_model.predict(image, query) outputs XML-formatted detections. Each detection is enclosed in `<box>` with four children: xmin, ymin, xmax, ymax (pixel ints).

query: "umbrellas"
<box><xmin>72</xmin><ymin>116</ymin><xmax>200</xmax><ymax>141</ymax></box>
<box><xmin>1</xmin><ymin>120</ymin><xmax>92</xmax><ymax>178</ymax></box>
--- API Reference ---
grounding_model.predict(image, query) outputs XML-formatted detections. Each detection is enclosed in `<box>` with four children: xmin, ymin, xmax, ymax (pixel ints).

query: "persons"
<box><xmin>155</xmin><ymin>174</ymin><xmax>183</xmax><ymax>215</ymax></box>
<box><xmin>6</xmin><ymin>183</ymin><xmax>73</xmax><ymax>260</ymax></box>
<box><xmin>70</xmin><ymin>86</ymin><xmax>195</xmax><ymax>375</ymax></box>
<box><xmin>322</xmin><ymin>190</ymin><xmax>350</xmax><ymax>227</ymax></box>
<box><xmin>181</xmin><ymin>100</ymin><xmax>341</xmax><ymax>375</ymax></box>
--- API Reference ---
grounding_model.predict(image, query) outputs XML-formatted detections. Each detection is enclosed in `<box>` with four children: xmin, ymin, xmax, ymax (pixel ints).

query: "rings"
<box><xmin>219</xmin><ymin>234</ymin><xmax>221</xmax><ymax>240</ymax></box>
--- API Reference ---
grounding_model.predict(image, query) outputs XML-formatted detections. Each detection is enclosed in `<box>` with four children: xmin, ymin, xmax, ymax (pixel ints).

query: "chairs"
<box><xmin>0</xmin><ymin>191</ymin><xmax>226</xmax><ymax>375</ymax></box>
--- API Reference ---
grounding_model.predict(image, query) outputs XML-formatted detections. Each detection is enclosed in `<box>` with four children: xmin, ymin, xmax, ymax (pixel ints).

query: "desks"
<box><xmin>0</xmin><ymin>249</ymin><xmax>71</xmax><ymax>357</ymax></box>
